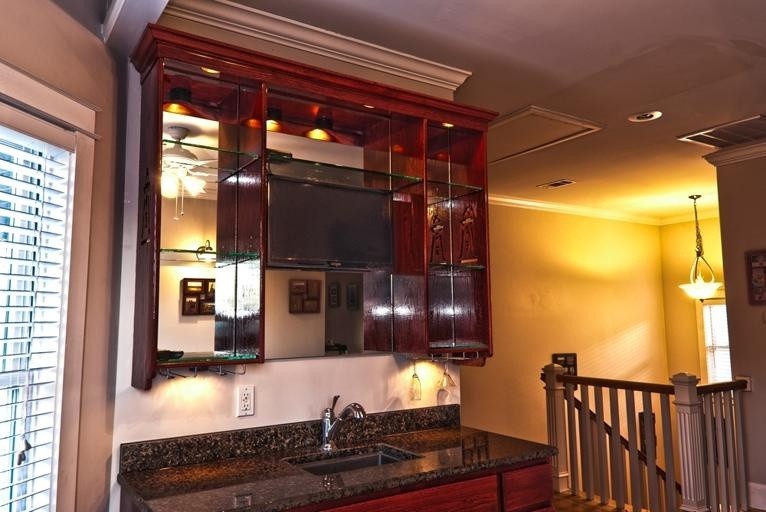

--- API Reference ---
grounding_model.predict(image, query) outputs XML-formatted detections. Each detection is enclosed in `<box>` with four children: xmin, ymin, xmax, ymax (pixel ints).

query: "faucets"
<box><xmin>320</xmin><ymin>473</ymin><xmax>345</xmax><ymax>500</ymax></box>
<box><xmin>320</xmin><ymin>393</ymin><xmax>367</xmax><ymax>451</ymax></box>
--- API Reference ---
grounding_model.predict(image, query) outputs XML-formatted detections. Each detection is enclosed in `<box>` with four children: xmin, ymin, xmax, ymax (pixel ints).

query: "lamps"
<box><xmin>677</xmin><ymin>194</ymin><xmax>722</xmax><ymax>302</ymax></box>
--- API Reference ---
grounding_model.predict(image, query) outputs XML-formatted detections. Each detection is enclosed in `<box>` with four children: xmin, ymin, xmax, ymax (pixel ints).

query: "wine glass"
<box><xmin>433</xmin><ymin>353</ymin><xmax>455</xmax><ymax>391</ymax></box>
<box><xmin>408</xmin><ymin>358</ymin><xmax>421</xmax><ymax>403</ymax></box>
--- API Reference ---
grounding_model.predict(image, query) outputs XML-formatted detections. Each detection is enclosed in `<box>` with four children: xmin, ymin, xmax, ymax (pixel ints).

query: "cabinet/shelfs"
<box><xmin>126</xmin><ymin>20</ymin><xmax>499</xmax><ymax>391</ymax></box>
<box><xmin>296</xmin><ymin>455</ymin><xmax>555</xmax><ymax>512</ymax></box>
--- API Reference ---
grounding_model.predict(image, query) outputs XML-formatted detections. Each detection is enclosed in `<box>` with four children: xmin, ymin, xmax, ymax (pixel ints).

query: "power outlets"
<box><xmin>236</xmin><ymin>384</ymin><xmax>254</xmax><ymax>416</ymax></box>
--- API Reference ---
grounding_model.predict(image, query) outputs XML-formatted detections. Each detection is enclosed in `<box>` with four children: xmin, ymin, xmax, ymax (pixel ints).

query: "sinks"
<box><xmin>281</xmin><ymin>442</ymin><xmax>427</xmax><ymax>478</ymax></box>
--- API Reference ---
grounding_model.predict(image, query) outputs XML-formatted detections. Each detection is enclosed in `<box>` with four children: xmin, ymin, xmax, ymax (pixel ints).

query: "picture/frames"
<box><xmin>744</xmin><ymin>249</ymin><xmax>766</xmax><ymax>305</ymax></box>
